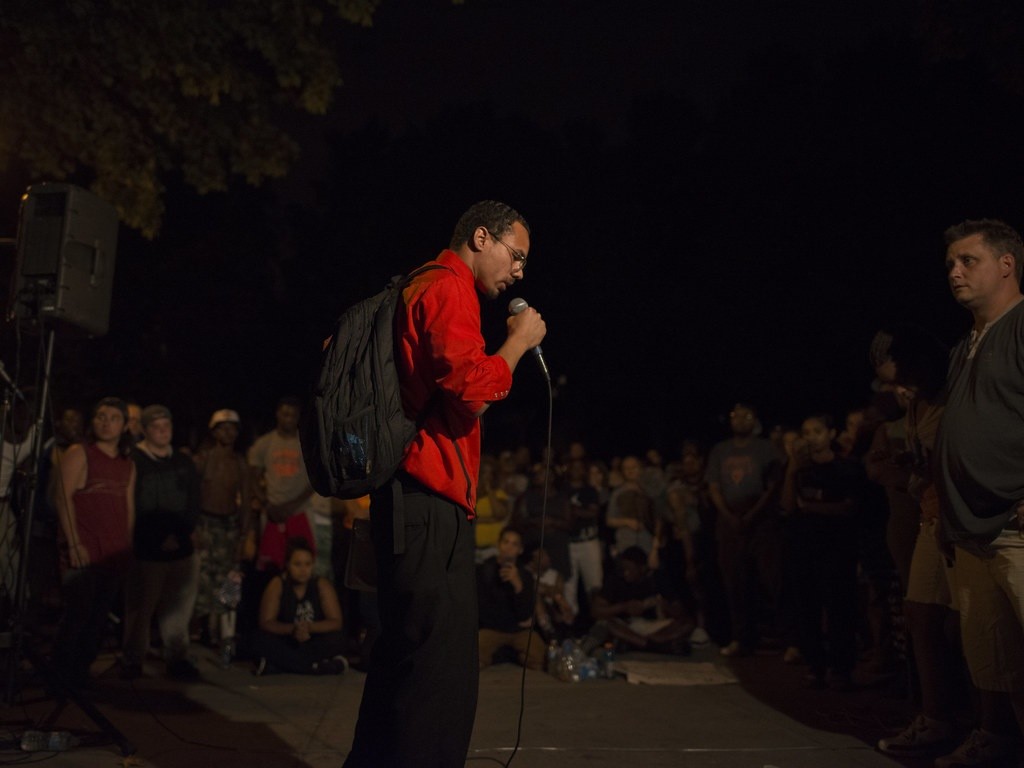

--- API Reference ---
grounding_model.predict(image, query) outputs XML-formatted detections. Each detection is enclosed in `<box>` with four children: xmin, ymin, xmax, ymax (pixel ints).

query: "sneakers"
<box><xmin>879</xmin><ymin>715</ymin><xmax>960</xmax><ymax>758</ymax></box>
<box><xmin>934</xmin><ymin>727</ymin><xmax>1009</xmax><ymax>768</ymax></box>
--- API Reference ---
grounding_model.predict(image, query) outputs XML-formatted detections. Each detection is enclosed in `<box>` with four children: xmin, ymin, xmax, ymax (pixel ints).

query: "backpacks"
<box><xmin>298</xmin><ymin>264</ymin><xmax>456</xmax><ymax>500</ymax></box>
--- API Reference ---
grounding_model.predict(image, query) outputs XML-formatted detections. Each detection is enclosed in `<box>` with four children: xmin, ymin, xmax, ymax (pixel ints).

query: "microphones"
<box><xmin>509</xmin><ymin>298</ymin><xmax>552</xmax><ymax>382</ymax></box>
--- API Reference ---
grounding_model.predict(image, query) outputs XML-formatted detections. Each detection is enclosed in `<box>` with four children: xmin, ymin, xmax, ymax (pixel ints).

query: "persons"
<box><xmin>935</xmin><ymin>222</ymin><xmax>1024</xmax><ymax>767</ymax></box>
<box><xmin>345</xmin><ymin>201</ymin><xmax>546</xmax><ymax>768</ymax></box>
<box><xmin>0</xmin><ymin>395</ymin><xmax>375</xmax><ymax>679</ymax></box>
<box><xmin>473</xmin><ymin>358</ymin><xmax>975</xmax><ymax>755</ymax></box>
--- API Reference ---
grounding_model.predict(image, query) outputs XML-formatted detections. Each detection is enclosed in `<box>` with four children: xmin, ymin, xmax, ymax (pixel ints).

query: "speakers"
<box><xmin>10</xmin><ymin>182</ymin><xmax>119</xmax><ymax>340</ymax></box>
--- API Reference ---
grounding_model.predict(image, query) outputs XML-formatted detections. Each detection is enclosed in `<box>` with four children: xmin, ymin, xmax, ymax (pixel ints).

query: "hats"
<box><xmin>209</xmin><ymin>409</ymin><xmax>241</xmax><ymax>431</ymax></box>
<box><xmin>142</xmin><ymin>404</ymin><xmax>172</xmax><ymax>426</ymax></box>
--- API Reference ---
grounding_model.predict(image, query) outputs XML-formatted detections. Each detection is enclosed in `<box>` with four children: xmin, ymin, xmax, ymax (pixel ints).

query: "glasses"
<box><xmin>729</xmin><ymin>412</ymin><xmax>754</xmax><ymax>421</ymax></box>
<box><xmin>487</xmin><ymin>231</ymin><xmax>527</xmax><ymax>272</ymax></box>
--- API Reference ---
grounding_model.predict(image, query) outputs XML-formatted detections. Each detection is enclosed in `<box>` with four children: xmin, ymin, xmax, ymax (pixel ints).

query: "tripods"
<box><xmin>0</xmin><ymin>330</ymin><xmax>137</xmax><ymax>756</ymax></box>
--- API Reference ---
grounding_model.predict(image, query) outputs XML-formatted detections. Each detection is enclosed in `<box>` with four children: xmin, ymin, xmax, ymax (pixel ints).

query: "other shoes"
<box><xmin>313</xmin><ymin>656</ymin><xmax>349</xmax><ymax>675</ymax></box>
<box><xmin>802</xmin><ymin>662</ymin><xmax>850</xmax><ymax>688</ymax></box>
<box><xmin>256</xmin><ymin>658</ymin><xmax>278</xmax><ymax>676</ymax></box>
<box><xmin>617</xmin><ymin>626</ymin><xmax>801</xmax><ymax>663</ymax></box>
<box><xmin>166</xmin><ymin>662</ymin><xmax>206</xmax><ymax>682</ymax></box>
<box><xmin>75</xmin><ymin>677</ymin><xmax>114</xmax><ymax>693</ymax></box>
<box><xmin>119</xmin><ymin>661</ymin><xmax>139</xmax><ymax>679</ymax></box>
